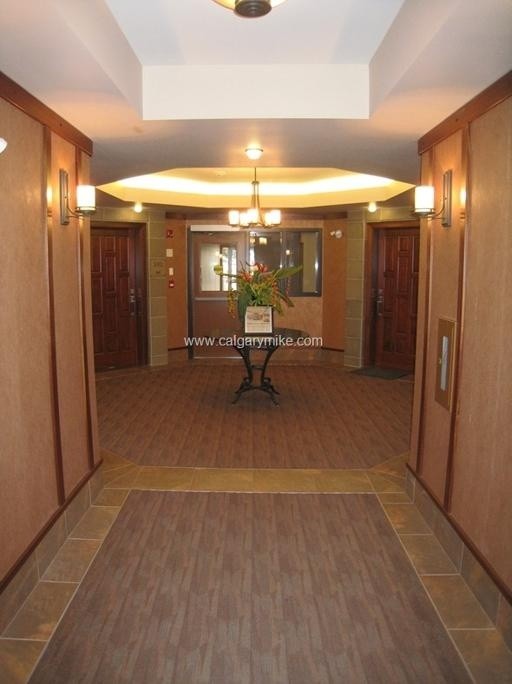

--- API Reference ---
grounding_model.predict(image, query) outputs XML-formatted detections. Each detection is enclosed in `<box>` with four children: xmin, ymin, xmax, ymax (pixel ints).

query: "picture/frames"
<box><xmin>243</xmin><ymin>304</ymin><xmax>275</xmax><ymax>335</ymax></box>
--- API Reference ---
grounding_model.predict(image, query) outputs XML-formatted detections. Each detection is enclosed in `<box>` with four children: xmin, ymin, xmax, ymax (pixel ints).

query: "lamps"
<box><xmin>411</xmin><ymin>168</ymin><xmax>452</xmax><ymax>227</ymax></box>
<box><xmin>246</xmin><ymin>147</ymin><xmax>263</xmax><ymax>160</ymax></box>
<box><xmin>227</xmin><ymin>168</ymin><xmax>282</xmax><ymax>230</ymax></box>
<box><xmin>59</xmin><ymin>167</ymin><xmax>97</xmax><ymax>224</ymax></box>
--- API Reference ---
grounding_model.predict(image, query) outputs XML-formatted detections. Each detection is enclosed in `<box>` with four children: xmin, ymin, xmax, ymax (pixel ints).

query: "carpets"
<box><xmin>350</xmin><ymin>365</ymin><xmax>411</xmax><ymax>380</ymax></box>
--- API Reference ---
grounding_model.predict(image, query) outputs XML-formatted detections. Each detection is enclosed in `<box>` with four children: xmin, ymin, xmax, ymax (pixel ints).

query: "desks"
<box><xmin>203</xmin><ymin>329</ymin><xmax>311</xmax><ymax>407</ymax></box>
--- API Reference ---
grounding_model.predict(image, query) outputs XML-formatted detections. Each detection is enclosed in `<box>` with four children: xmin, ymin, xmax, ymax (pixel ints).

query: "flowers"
<box><xmin>214</xmin><ymin>259</ymin><xmax>304</xmax><ymax>334</ymax></box>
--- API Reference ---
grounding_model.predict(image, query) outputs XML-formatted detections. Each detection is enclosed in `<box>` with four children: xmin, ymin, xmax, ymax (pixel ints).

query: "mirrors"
<box><xmin>187</xmin><ymin>228</ymin><xmax>326</xmax><ymax>299</ymax></box>
